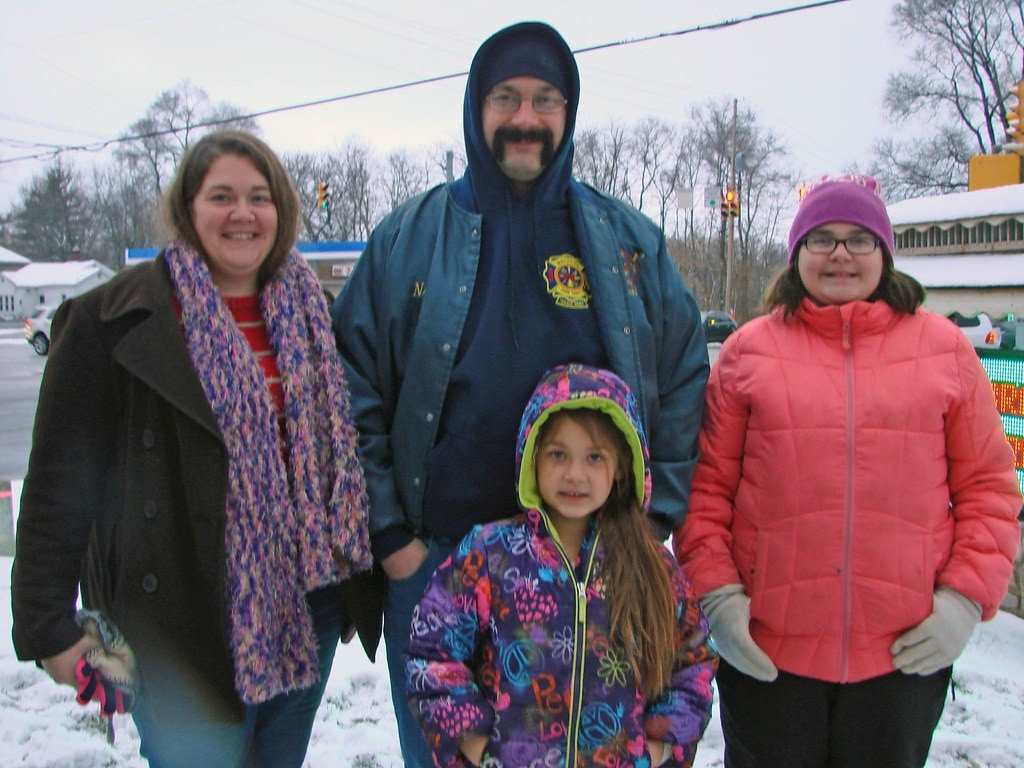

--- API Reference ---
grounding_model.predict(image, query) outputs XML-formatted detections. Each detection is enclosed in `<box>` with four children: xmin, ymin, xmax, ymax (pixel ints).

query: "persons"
<box><xmin>672</xmin><ymin>180</ymin><xmax>1024</xmax><ymax>768</ymax></box>
<box><xmin>328</xmin><ymin>22</ymin><xmax>711</xmax><ymax>767</ymax></box>
<box><xmin>10</xmin><ymin>129</ymin><xmax>384</xmax><ymax>768</ymax></box>
<box><xmin>403</xmin><ymin>364</ymin><xmax>748</xmax><ymax>768</ymax></box>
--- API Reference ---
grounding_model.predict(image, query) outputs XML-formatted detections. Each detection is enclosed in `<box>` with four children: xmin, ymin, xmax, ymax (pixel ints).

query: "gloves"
<box><xmin>74</xmin><ymin>659</ymin><xmax>129</xmax><ymax>715</ymax></box>
<box><xmin>697</xmin><ymin>584</ymin><xmax>779</xmax><ymax>681</ymax></box>
<box><xmin>888</xmin><ymin>585</ymin><xmax>982</xmax><ymax>676</ymax></box>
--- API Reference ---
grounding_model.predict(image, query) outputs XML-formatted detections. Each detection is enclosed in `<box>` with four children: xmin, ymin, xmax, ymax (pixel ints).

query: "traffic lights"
<box><xmin>721</xmin><ymin>191</ymin><xmax>739</xmax><ymax>217</ymax></box>
<box><xmin>318</xmin><ymin>182</ymin><xmax>330</xmax><ymax>210</ymax></box>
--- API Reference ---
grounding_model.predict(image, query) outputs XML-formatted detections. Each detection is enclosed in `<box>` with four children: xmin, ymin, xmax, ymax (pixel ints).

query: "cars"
<box><xmin>24</xmin><ymin>305</ymin><xmax>58</xmax><ymax>355</ymax></box>
<box><xmin>947</xmin><ymin>312</ymin><xmax>1003</xmax><ymax>349</ymax></box>
<box><xmin>701</xmin><ymin>308</ymin><xmax>740</xmax><ymax>345</ymax></box>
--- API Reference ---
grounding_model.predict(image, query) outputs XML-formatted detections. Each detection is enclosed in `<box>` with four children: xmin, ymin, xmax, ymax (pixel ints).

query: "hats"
<box><xmin>478</xmin><ymin>31</ymin><xmax>571</xmax><ymax>99</ymax></box>
<box><xmin>789</xmin><ymin>174</ymin><xmax>895</xmax><ymax>264</ymax></box>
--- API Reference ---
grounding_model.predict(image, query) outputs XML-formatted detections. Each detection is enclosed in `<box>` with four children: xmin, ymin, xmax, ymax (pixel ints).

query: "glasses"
<box><xmin>800</xmin><ymin>231</ymin><xmax>884</xmax><ymax>255</ymax></box>
<box><xmin>481</xmin><ymin>94</ymin><xmax>567</xmax><ymax>114</ymax></box>
